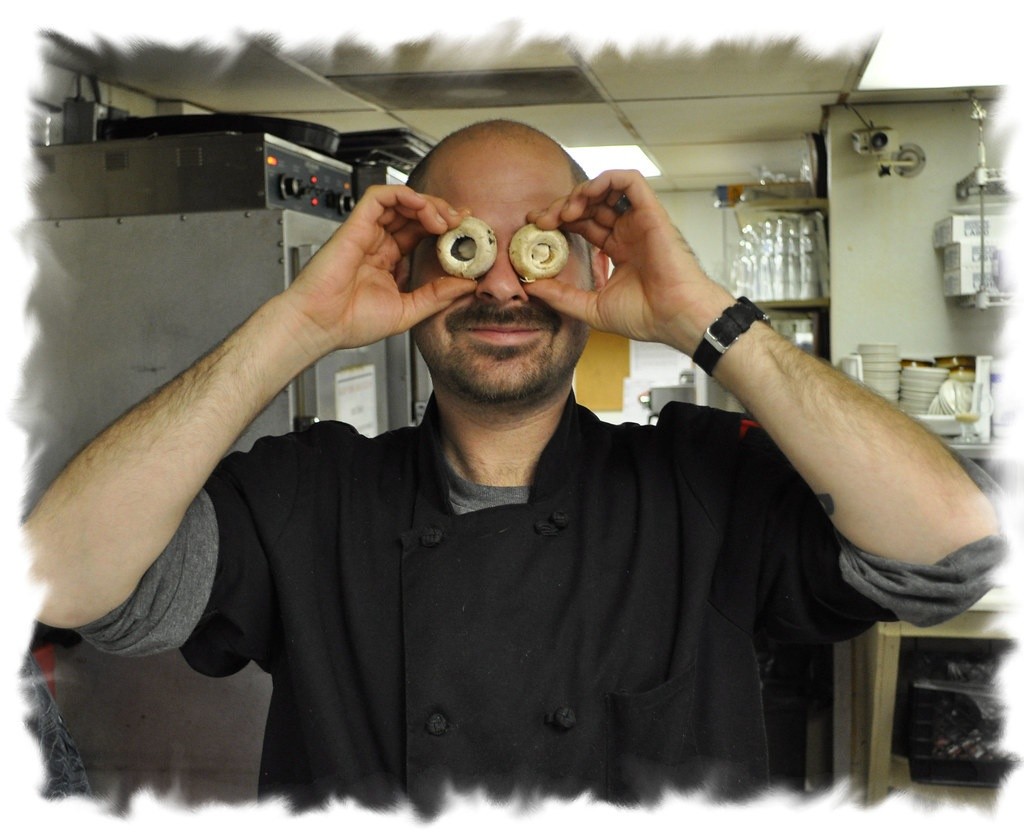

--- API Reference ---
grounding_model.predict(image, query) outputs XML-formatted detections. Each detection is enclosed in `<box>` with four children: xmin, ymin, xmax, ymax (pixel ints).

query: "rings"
<box><xmin>614</xmin><ymin>193</ymin><xmax>631</xmax><ymax>214</ymax></box>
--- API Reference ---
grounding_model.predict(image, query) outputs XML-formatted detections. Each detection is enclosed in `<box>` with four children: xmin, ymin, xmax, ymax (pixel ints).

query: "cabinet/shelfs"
<box><xmin>749</xmin><ymin>198</ymin><xmax>831</xmax><ymax>318</ymax></box>
<box><xmin>955</xmin><ymin>89</ymin><xmax>1015</xmax><ymax>310</ymax></box>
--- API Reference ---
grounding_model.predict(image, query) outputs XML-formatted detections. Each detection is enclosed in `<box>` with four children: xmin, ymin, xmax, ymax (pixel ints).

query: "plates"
<box><xmin>899</xmin><ymin>365</ymin><xmax>949</xmax><ymax>412</ymax></box>
<box><xmin>927</xmin><ymin>379</ymin><xmax>956</xmax><ymax>418</ymax></box>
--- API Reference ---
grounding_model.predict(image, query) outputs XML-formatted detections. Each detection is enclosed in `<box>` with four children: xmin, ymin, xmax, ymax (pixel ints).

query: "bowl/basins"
<box><xmin>948</xmin><ymin>365</ymin><xmax>980</xmax><ymax>379</ymax></box>
<box><xmin>901</xmin><ymin>359</ymin><xmax>934</xmax><ymax>370</ymax></box>
<box><xmin>859</xmin><ymin>344</ymin><xmax>900</xmax><ymax>403</ymax></box>
<box><xmin>637</xmin><ymin>385</ymin><xmax>696</xmax><ymax>415</ymax></box>
<box><xmin>937</xmin><ymin>354</ymin><xmax>978</xmax><ymax>365</ymax></box>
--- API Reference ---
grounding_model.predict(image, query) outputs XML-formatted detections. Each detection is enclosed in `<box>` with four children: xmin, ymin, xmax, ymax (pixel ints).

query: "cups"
<box><xmin>730</xmin><ymin>218</ymin><xmax>817</xmax><ymax>302</ymax></box>
<box><xmin>954</xmin><ymin>381</ymin><xmax>994</xmax><ymax>443</ymax></box>
<box><xmin>843</xmin><ymin>353</ymin><xmax>862</xmax><ymax>383</ymax></box>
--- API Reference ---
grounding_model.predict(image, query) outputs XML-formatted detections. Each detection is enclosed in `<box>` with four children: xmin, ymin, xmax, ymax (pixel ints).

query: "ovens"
<box><xmin>30</xmin><ymin>133</ymin><xmax>392</xmax><ymax>804</ymax></box>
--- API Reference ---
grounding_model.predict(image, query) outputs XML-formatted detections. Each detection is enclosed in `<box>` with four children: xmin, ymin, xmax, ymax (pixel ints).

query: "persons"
<box><xmin>26</xmin><ymin>120</ymin><xmax>1009</xmax><ymax>816</ymax></box>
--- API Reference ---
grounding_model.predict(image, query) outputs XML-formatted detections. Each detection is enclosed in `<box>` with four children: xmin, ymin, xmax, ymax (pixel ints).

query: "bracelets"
<box><xmin>692</xmin><ymin>295</ymin><xmax>771</xmax><ymax>377</ymax></box>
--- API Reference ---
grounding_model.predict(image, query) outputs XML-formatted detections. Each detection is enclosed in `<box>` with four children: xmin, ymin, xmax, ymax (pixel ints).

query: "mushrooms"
<box><xmin>508</xmin><ymin>223</ymin><xmax>569</xmax><ymax>282</ymax></box>
<box><xmin>435</xmin><ymin>216</ymin><xmax>498</xmax><ymax>279</ymax></box>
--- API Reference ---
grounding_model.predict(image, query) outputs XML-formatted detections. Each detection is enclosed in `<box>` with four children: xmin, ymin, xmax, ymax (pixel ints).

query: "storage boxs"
<box><xmin>934</xmin><ymin>214</ymin><xmax>1008</xmax><ymax>249</ymax></box>
<box><xmin>945</xmin><ymin>242</ymin><xmax>999</xmax><ymax>271</ymax></box>
<box><xmin>943</xmin><ymin>271</ymin><xmax>1000</xmax><ymax>299</ymax></box>
